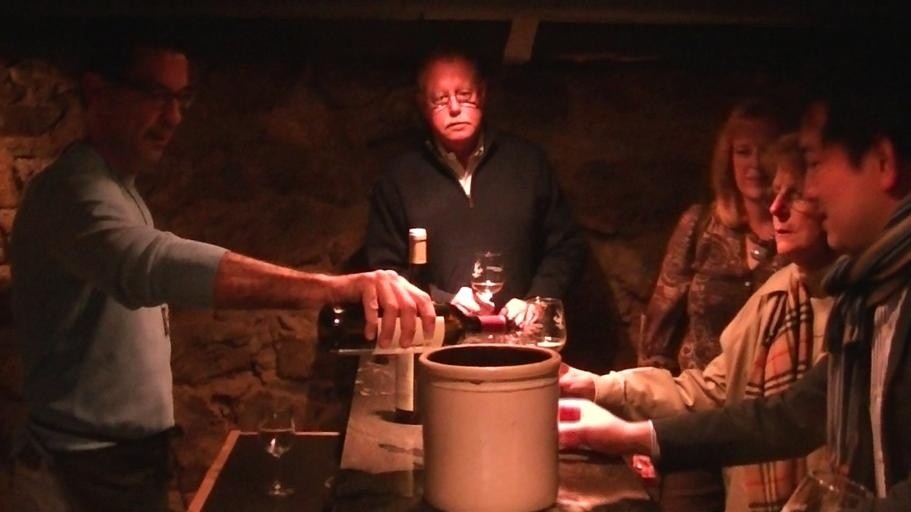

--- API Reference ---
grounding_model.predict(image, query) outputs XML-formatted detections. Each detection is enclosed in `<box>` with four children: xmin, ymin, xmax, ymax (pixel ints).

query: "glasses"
<box><xmin>429</xmin><ymin>89</ymin><xmax>474</xmax><ymax>105</ymax></box>
<box><xmin>767</xmin><ymin>186</ymin><xmax>817</xmax><ymax>214</ymax></box>
<box><xmin>135</xmin><ymin>82</ymin><xmax>195</xmax><ymax>110</ymax></box>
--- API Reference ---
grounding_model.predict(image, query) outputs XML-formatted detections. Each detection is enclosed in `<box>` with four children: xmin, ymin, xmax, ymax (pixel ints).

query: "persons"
<box><xmin>361</xmin><ymin>44</ymin><xmax>589</xmax><ymax>333</ymax></box>
<box><xmin>556</xmin><ymin>130</ymin><xmax>837</xmax><ymax>512</ymax></box>
<box><xmin>556</xmin><ymin>83</ymin><xmax>909</xmax><ymax>511</ymax></box>
<box><xmin>0</xmin><ymin>28</ymin><xmax>437</xmax><ymax>512</ymax></box>
<box><xmin>630</xmin><ymin>102</ymin><xmax>795</xmax><ymax>511</ymax></box>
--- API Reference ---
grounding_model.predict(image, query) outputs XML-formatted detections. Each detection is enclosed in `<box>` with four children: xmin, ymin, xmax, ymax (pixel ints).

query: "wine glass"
<box><xmin>471</xmin><ymin>254</ymin><xmax>503</xmax><ymax>323</ymax></box>
<box><xmin>783</xmin><ymin>470</ymin><xmax>874</xmax><ymax>512</ymax></box>
<box><xmin>521</xmin><ymin>298</ymin><xmax>565</xmax><ymax>357</ymax></box>
<box><xmin>256</xmin><ymin>406</ymin><xmax>299</xmax><ymax>499</ymax></box>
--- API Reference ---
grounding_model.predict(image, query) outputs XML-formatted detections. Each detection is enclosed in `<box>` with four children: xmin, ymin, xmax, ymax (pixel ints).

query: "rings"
<box><xmin>634</xmin><ymin>463</ymin><xmax>643</xmax><ymax>471</ymax></box>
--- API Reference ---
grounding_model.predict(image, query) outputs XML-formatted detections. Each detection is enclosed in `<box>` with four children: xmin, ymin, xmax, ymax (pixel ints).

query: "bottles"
<box><xmin>394</xmin><ymin>226</ymin><xmax>444</xmax><ymax>420</ymax></box>
<box><xmin>316</xmin><ymin>304</ymin><xmax>476</xmax><ymax>352</ymax></box>
